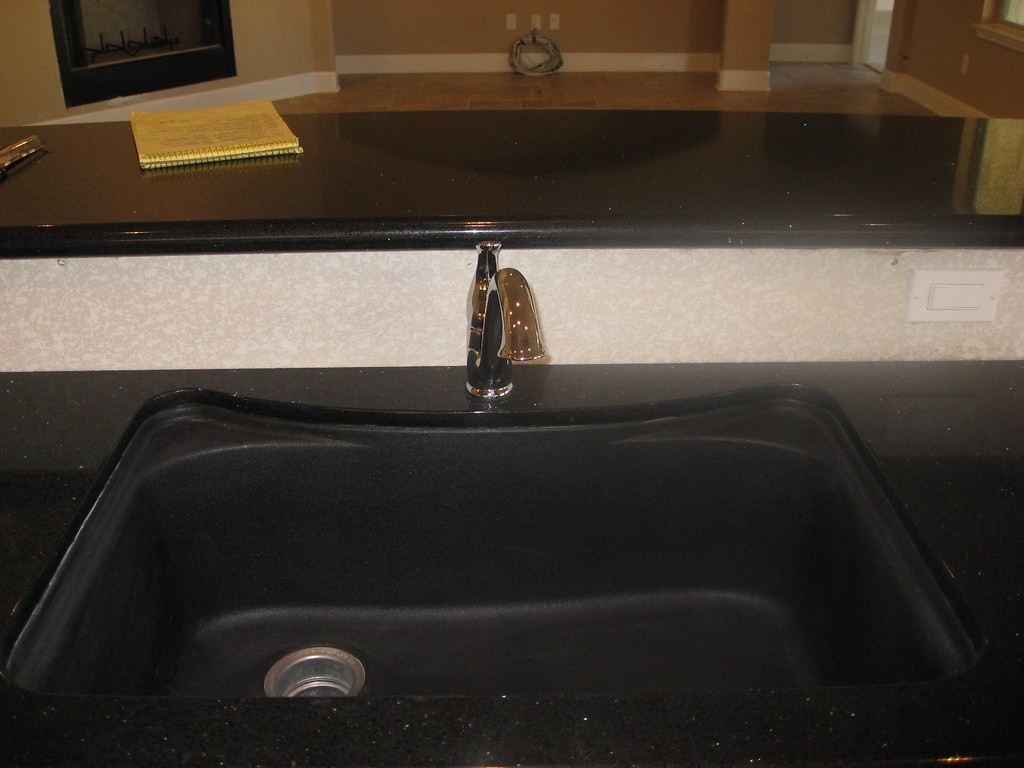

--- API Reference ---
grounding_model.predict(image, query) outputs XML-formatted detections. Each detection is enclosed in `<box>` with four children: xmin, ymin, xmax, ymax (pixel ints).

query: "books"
<box><xmin>126</xmin><ymin>96</ymin><xmax>302</xmax><ymax>169</ymax></box>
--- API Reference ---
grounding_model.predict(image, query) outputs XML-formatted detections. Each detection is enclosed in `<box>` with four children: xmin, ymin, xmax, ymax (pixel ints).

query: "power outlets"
<box><xmin>506</xmin><ymin>14</ymin><xmax>516</xmax><ymax>30</ymax></box>
<box><xmin>549</xmin><ymin>14</ymin><xmax>559</xmax><ymax>30</ymax></box>
<box><xmin>530</xmin><ymin>14</ymin><xmax>541</xmax><ymax>29</ymax></box>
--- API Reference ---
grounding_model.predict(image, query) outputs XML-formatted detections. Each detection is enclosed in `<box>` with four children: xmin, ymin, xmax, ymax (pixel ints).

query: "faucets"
<box><xmin>465</xmin><ymin>240</ymin><xmax>547</xmax><ymax>400</ymax></box>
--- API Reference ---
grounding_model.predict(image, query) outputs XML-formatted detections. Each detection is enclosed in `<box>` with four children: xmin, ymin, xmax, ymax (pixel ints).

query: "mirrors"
<box><xmin>0</xmin><ymin>0</ymin><xmax>1024</xmax><ymax>251</ymax></box>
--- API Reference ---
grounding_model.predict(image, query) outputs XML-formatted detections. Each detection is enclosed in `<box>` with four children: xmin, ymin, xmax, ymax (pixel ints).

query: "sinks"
<box><xmin>1</xmin><ymin>380</ymin><xmax>999</xmax><ymax>702</ymax></box>
